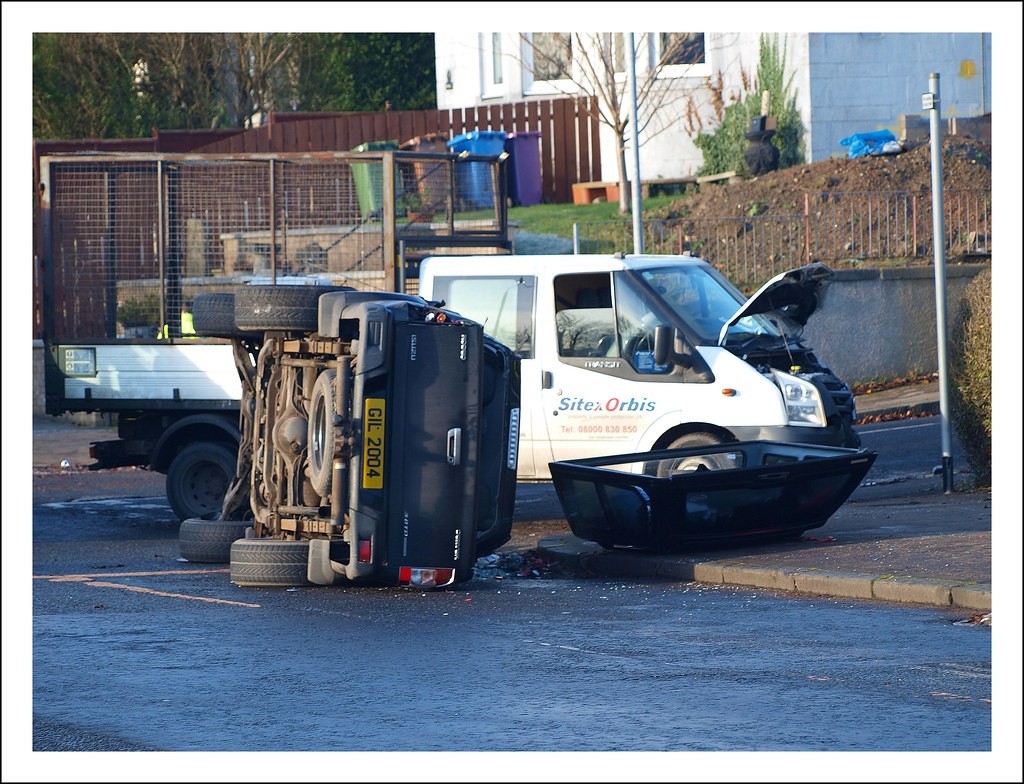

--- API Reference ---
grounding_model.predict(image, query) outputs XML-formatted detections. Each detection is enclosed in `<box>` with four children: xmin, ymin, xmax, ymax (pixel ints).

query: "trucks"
<box><xmin>37</xmin><ymin>147</ymin><xmax>865</xmax><ymax>487</ymax></box>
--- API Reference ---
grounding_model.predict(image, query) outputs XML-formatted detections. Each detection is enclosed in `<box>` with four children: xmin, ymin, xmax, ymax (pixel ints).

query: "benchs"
<box><xmin>571</xmin><ymin>169</ymin><xmax>743</xmax><ymax>204</ymax></box>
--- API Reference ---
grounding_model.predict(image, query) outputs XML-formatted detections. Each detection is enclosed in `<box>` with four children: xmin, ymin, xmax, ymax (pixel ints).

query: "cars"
<box><xmin>176</xmin><ymin>283</ymin><xmax>523</xmax><ymax>592</ymax></box>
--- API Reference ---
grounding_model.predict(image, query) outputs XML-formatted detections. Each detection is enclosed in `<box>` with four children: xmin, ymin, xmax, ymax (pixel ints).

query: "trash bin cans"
<box><xmin>347</xmin><ymin>138</ymin><xmax>406</xmax><ymax>224</ymax></box>
<box><xmin>397</xmin><ymin>129</ymin><xmax>456</xmax><ymax>214</ymax></box>
<box><xmin>505</xmin><ymin>131</ymin><xmax>543</xmax><ymax>207</ymax></box>
<box><xmin>446</xmin><ymin>130</ymin><xmax>508</xmax><ymax>212</ymax></box>
